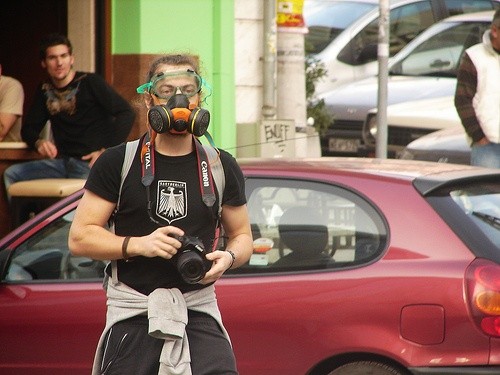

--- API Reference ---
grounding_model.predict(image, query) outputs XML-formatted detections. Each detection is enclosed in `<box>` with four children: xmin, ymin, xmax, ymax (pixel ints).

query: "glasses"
<box><xmin>136</xmin><ymin>70</ymin><xmax>203</xmax><ymax>99</ymax></box>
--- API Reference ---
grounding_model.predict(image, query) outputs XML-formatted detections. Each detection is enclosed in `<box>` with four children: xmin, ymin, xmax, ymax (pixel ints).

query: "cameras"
<box><xmin>171</xmin><ymin>234</ymin><xmax>216</xmax><ymax>291</ymax></box>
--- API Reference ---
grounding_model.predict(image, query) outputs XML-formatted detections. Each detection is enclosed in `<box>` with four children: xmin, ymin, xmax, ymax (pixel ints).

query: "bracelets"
<box><xmin>223</xmin><ymin>251</ymin><xmax>235</xmax><ymax>274</ymax></box>
<box><xmin>122</xmin><ymin>236</ymin><xmax>134</xmax><ymax>262</ymax></box>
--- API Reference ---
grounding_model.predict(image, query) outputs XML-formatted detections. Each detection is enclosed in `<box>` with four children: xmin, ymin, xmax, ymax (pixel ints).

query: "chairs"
<box><xmin>268</xmin><ymin>207</ymin><xmax>334</xmax><ymax>268</ymax></box>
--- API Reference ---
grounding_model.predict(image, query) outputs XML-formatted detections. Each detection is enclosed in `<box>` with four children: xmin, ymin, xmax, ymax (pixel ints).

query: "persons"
<box><xmin>3</xmin><ymin>39</ymin><xmax>133</xmax><ymax>214</ymax></box>
<box><xmin>454</xmin><ymin>10</ymin><xmax>500</xmax><ymax>168</ymax></box>
<box><xmin>68</xmin><ymin>55</ymin><xmax>253</xmax><ymax>375</ymax></box>
<box><xmin>0</xmin><ymin>61</ymin><xmax>24</xmax><ymax>142</ymax></box>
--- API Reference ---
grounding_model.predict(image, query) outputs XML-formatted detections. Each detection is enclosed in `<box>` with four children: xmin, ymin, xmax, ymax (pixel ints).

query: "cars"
<box><xmin>308</xmin><ymin>0</ymin><xmax>500</xmax><ymax>106</ymax></box>
<box><xmin>307</xmin><ymin>9</ymin><xmax>498</xmax><ymax>157</ymax></box>
<box><xmin>0</xmin><ymin>159</ymin><xmax>500</xmax><ymax>375</ymax></box>
<box><xmin>403</xmin><ymin>126</ymin><xmax>476</xmax><ymax>165</ymax></box>
<box><xmin>363</xmin><ymin>97</ymin><xmax>464</xmax><ymax>158</ymax></box>
